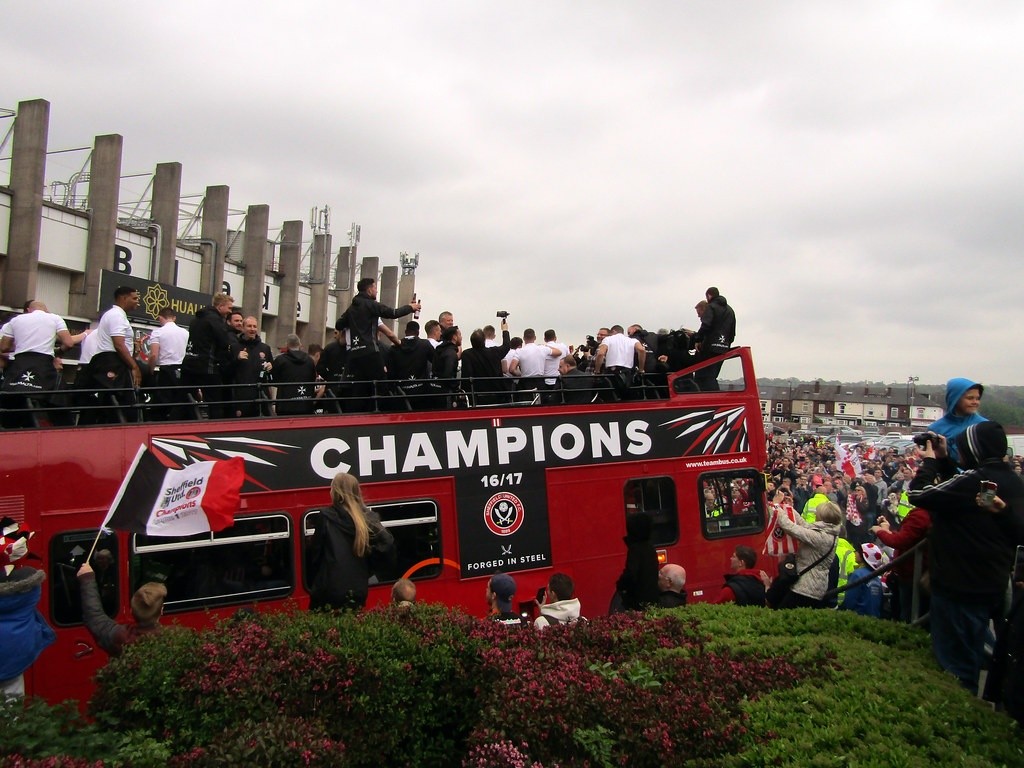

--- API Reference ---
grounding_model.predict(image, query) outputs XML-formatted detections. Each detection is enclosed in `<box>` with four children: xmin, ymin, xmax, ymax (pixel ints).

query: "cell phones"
<box><xmin>981</xmin><ymin>480</ymin><xmax>997</xmax><ymax>507</ymax></box>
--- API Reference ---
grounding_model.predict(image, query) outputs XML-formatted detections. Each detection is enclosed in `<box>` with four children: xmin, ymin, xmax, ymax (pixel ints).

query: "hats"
<box><xmin>491</xmin><ymin>574</ymin><xmax>515</xmax><ymax>612</ymax></box>
<box><xmin>861</xmin><ymin>543</ymin><xmax>882</xmax><ymax>570</ymax></box>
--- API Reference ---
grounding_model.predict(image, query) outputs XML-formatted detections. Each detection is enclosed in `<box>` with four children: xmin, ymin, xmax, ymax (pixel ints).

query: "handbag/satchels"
<box><xmin>777</xmin><ymin>553</ymin><xmax>799</xmax><ymax>587</ymax></box>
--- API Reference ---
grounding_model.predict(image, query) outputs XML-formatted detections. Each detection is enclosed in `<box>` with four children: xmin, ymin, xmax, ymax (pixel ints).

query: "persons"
<box><xmin>532</xmin><ymin>573</ymin><xmax>588</xmax><ymax>630</ymax></box>
<box><xmin>0</xmin><ymin>278</ymin><xmax>736</xmax><ymax>430</ymax></box>
<box><xmin>0</xmin><ymin>517</ymin><xmax>56</xmax><ymax>699</ymax></box>
<box><xmin>304</xmin><ymin>473</ymin><xmax>393</xmax><ymax>614</ymax></box>
<box><xmin>392</xmin><ymin>579</ymin><xmax>415</xmax><ymax>606</ymax></box>
<box><xmin>616</xmin><ymin>563</ymin><xmax>687</xmax><ymax>609</ymax></box>
<box><xmin>702</xmin><ymin>427</ymin><xmax>1024</xmax><ymax>722</ymax></box>
<box><xmin>925</xmin><ymin>378</ymin><xmax>991</xmax><ymax>464</ymax></box>
<box><xmin>78</xmin><ymin>563</ymin><xmax>168</xmax><ymax>661</ymax></box>
<box><xmin>484</xmin><ymin>573</ymin><xmax>523</xmax><ymax>626</ymax></box>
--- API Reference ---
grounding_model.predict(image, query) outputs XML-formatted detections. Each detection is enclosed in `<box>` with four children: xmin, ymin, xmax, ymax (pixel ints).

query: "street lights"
<box><xmin>909</xmin><ymin>376</ymin><xmax>919</xmax><ymax>427</ymax></box>
<box><xmin>787</xmin><ymin>381</ymin><xmax>792</xmax><ymax>415</ymax></box>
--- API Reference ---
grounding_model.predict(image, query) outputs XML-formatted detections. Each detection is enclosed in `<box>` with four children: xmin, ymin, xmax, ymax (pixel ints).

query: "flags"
<box><xmin>104</xmin><ymin>444</ymin><xmax>245</xmax><ymax>536</ymax></box>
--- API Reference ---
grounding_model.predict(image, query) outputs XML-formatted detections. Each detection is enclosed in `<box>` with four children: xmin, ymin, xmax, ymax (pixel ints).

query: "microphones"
<box><xmin>496</xmin><ymin>310</ymin><xmax>510</xmax><ymax>317</ymax></box>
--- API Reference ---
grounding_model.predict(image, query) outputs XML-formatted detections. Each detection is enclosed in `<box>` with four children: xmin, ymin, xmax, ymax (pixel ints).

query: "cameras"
<box><xmin>912</xmin><ymin>431</ymin><xmax>940</xmax><ymax>450</ymax></box>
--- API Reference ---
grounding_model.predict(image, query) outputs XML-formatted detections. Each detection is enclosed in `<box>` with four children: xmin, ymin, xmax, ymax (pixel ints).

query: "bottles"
<box><xmin>414</xmin><ymin>300</ymin><xmax>421</xmax><ymax>319</ymax></box>
<box><xmin>411</xmin><ymin>293</ymin><xmax>417</xmax><ymax>303</ymax></box>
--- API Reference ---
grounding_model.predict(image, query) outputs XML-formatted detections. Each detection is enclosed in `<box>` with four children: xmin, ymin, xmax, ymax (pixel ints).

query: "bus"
<box><xmin>0</xmin><ymin>348</ymin><xmax>780</xmax><ymax>736</ymax></box>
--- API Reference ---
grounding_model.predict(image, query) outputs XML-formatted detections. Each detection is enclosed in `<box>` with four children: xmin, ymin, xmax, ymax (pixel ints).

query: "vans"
<box><xmin>822</xmin><ymin>435</ymin><xmax>862</xmax><ymax>444</ymax></box>
